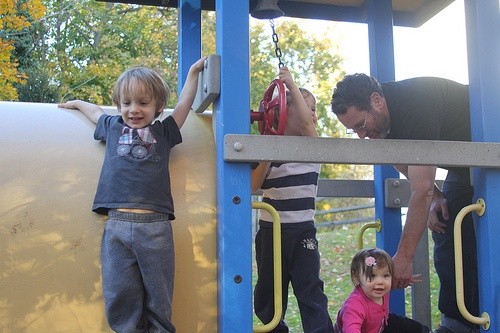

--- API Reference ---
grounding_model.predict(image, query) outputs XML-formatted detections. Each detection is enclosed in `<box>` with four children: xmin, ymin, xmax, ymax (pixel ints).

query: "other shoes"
<box><xmin>433</xmin><ymin>326</ymin><xmax>452</xmax><ymax>333</ymax></box>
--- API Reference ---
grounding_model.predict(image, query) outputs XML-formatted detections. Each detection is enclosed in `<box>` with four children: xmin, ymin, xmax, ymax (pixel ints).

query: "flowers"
<box><xmin>366</xmin><ymin>257</ymin><xmax>377</xmax><ymax>267</ymax></box>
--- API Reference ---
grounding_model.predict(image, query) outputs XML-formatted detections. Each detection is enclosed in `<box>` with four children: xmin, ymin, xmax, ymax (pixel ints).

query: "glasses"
<box><xmin>346</xmin><ymin>107</ymin><xmax>369</xmax><ymax>135</ymax></box>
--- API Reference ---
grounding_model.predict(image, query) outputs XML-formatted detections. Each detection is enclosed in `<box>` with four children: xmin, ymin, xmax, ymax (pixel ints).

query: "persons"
<box><xmin>334</xmin><ymin>249</ymin><xmax>423</xmax><ymax>333</ymax></box>
<box><xmin>331</xmin><ymin>73</ymin><xmax>480</xmax><ymax>333</ymax></box>
<box><xmin>57</xmin><ymin>52</ymin><xmax>208</xmax><ymax>333</ymax></box>
<box><xmin>251</xmin><ymin>66</ymin><xmax>335</xmax><ymax>333</ymax></box>
